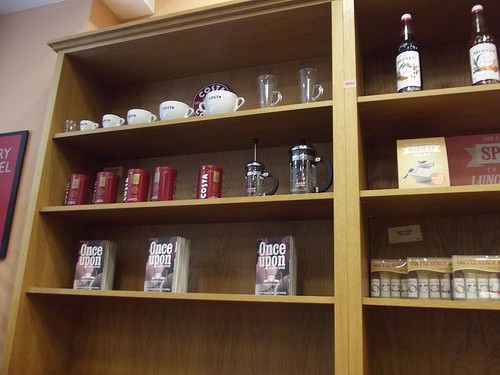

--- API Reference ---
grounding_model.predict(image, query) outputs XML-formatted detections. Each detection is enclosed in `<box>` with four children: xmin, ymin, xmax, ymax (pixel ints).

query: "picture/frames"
<box><xmin>0</xmin><ymin>130</ymin><xmax>29</xmax><ymax>259</ymax></box>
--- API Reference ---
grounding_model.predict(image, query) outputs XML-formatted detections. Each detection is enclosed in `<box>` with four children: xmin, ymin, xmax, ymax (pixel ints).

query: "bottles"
<box><xmin>371</xmin><ymin>268</ymin><xmax>500</xmax><ymax>299</ymax></box>
<box><xmin>396</xmin><ymin>14</ymin><xmax>423</xmax><ymax>93</ymax></box>
<box><xmin>469</xmin><ymin>4</ymin><xmax>500</xmax><ymax>86</ymax></box>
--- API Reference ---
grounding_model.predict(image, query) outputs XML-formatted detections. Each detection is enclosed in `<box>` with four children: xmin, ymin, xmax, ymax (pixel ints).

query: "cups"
<box><xmin>123</xmin><ymin>169</ymin><xmax>148</xmax><ymax>202</ymax></box>
<box><xmin>256</xmin><ymin>74</ymin><xmax>282</xmax><ymax>108</ymax></box>
<box><xmin>199</xmin><ymin>91</ymin><xmax>245</xmax><ymax>115</ymax></box>
<box><xmin>287</xmin><ymin>138</ymin><xmax>333</xmax><ymax>194</ymax></box>
<box><xmin>80</xmin><ymin>119</ymin><xmax>99</xmax><ymax>130</ymax></box>
<box><xmin>92</xmin><ymin>172</ymin><xmax>120</xmax><ymax>204</ymax></box>
<box><xmin>197</xmin><ymin>165</ymin><xmax>223</xmax><ymax>198</ymax></box>
<box><xmin>159</xmin><ymin>101</ymin><xmax>194</xmax><ymax>121</ymax></box>
<box><xmin>102</xmin><ymin>114</ymin><xmax>125</xmax><ymax>128</ymax></box>
<box><xmin>64</xmin><ymin>119</ymin><xmax>77</xmax><ymax>132</ymax></box>
<box><xmin>150</xmin><ymin>167</ymin><xmax>178</xmax><ymax>201</ymax></box>
<box><xmin>127</xmin><ymin>109</ymin><xmax>157</xmax><ymax>125</ymax></box>
<box><xmin>243</xmin><ymin>139</ymin><xmax>279</xmax><ymax>197</ymax></box>
<box><xmin>297</xmin><ymin>68</ymin><xmax>323</xmax><ymax>103</ymax></box>
<box><xmin>63</xmin><ymin>173</ymin><xmax>91</xmax><ymax>205</ymax></box>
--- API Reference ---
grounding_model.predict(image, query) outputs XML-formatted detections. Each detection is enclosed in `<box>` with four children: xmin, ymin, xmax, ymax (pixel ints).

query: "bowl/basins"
<box><xmin>411</xmin><ymin>162</ymin><xmax>440</xmax><ymax>182</ymax></box>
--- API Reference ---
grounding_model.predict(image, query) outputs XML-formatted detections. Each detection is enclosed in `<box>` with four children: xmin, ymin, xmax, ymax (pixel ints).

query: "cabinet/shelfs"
<box><xmin>0</xmin><ymin>0</ymin><xmax>499</xmax><ymax>374</ymax></box>
